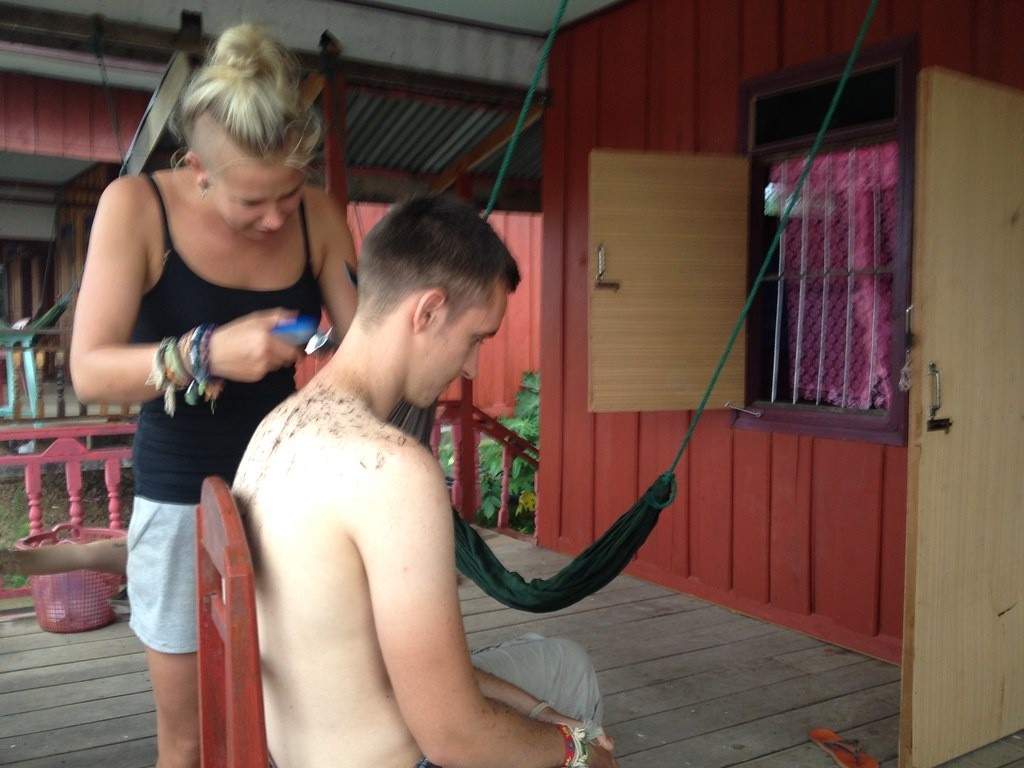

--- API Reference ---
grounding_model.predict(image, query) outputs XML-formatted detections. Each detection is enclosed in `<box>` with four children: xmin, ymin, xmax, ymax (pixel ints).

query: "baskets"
<box><xmin>15</xmin><ymin>521</ymin><xmax>128</xmax><ymax>634</ymax></box>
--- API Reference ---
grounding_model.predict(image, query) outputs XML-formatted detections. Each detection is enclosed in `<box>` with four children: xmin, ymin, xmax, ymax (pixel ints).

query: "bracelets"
<box><xmin>145</xmin><ymin>322</ymin><xmax>223</xmax><ymax>418</ymax></box>
<box><xmin>559</xmin><ymin>724</ymin><xmax>588</xmax><ymax>768</ymax></box>
<box><xmin>527</xmin><ymin>701</ymin><xmax>550</xmax><ymax>720</ymax></box>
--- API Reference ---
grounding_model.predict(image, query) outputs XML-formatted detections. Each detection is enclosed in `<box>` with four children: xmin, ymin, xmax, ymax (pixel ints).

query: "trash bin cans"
<box><xmin>14</xmin><ymin>523</ymin><xmax>128</xmax><ymax>633</ymax></box>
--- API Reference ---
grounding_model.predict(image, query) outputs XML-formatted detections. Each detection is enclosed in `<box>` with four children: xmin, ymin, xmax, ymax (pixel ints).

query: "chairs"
<box><xmin>196</xmin><ymin>476</ymin><xmax>272</xmax><ymax>768</ymax></box>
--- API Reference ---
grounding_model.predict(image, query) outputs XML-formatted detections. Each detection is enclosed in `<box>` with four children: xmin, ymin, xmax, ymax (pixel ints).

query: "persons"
<box><xmin>232</xmin><ymin>194</ymin><xmax>619</xmax><ymax>768</ymax></box>
<box><xmin>69</xmin><ymin>22</ymin><xmax>358</xmax><ymax>768</ymax></box>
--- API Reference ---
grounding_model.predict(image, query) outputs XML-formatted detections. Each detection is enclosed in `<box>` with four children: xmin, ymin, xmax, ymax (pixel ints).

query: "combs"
<box><xmin>343</xmin><ymin>261</ymin><xmax>357</xmax><ymax>286</ymax></box>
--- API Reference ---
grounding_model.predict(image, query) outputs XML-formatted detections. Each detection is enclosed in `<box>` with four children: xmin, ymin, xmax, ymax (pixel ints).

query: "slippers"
<box><xmin>809</xmin><ymin>728</ymin><xmax>879</xmax><ymax>768</ymax></box>
